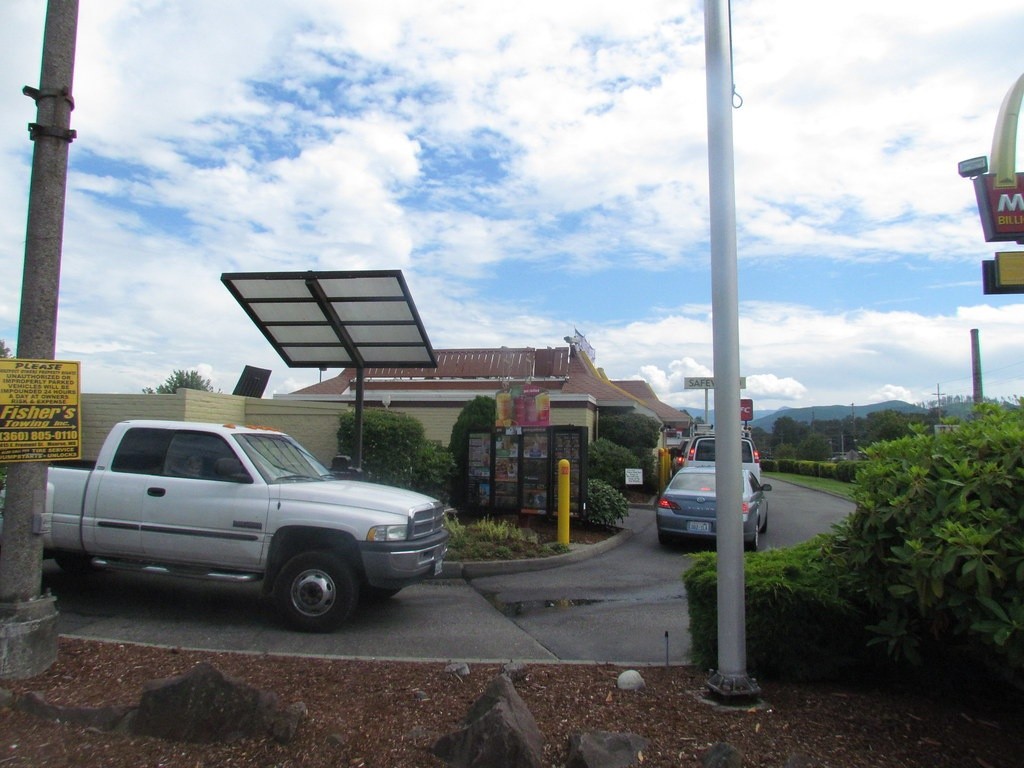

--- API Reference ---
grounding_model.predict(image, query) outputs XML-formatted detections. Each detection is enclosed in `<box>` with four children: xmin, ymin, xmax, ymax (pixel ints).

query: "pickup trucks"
<box><xmin>42</xmin><ymin>419</ymin><xmax>450</xmax><ymax>634</ymax></box>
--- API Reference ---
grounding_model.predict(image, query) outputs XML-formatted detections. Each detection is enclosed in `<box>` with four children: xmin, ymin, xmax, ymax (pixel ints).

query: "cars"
<box><xmin>656</xmin><ymin>468</ymin><xmax>772</xmax><ymax>551</ymax></box>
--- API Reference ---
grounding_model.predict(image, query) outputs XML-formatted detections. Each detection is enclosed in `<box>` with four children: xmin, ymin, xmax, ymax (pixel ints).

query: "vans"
<box><xmin>679</xmin><ymin>434</ymin><xmax>760</xmax><ymax>486</ymax></box>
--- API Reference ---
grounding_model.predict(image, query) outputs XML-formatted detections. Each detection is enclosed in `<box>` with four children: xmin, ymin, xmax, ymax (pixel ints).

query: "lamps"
<box><xmin>958</xmin><ymin>156</ymin><xmax>988</xmax><ymax>181</ymax></box>
